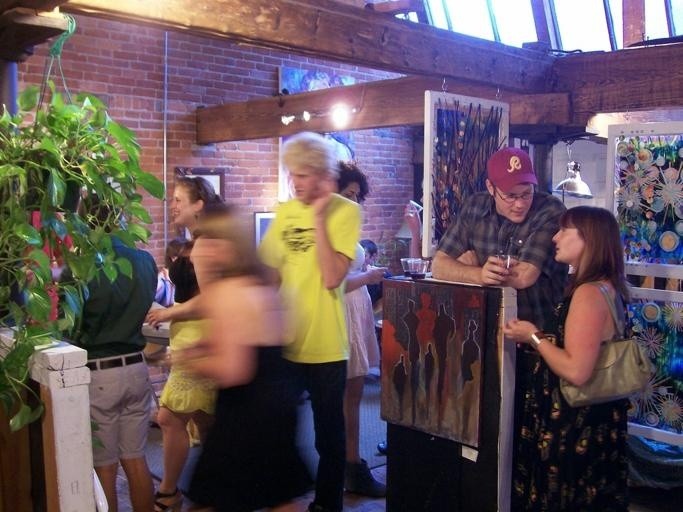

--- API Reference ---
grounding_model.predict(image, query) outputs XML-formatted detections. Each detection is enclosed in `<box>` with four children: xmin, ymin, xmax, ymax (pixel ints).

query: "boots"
<box><xmin>344</xmin><ymin>458</ymin><xmax>386</xmax><ymax>498</ymax></box>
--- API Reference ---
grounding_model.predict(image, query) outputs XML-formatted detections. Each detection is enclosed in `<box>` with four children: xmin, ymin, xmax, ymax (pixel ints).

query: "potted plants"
<box><xmin>0</xmin><ymin>80</ymin><xmax>165</xmax><ymax>435</ymax></box>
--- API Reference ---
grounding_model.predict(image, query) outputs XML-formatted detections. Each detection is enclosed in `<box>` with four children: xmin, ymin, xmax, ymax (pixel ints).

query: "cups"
<box><xmin>496</xmin><ymin>252</ymin><xmax>517</xmax><ymax>275</ymax></box>
<box><xmin>400</xmin><ymin>257</ymin><xmax>430</xmax><ymax>281</ymax></box>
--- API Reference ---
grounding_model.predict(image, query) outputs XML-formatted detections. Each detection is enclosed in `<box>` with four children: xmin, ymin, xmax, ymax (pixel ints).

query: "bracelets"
<box><xmin>528</xmin><ymin>330</ymin><xmax>548</xmax><ymax>351</ymax></box>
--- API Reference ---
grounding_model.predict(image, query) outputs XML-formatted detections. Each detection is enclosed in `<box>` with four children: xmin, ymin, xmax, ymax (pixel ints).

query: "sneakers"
<box><xmin>305</xmin><ymin>503</ymin><xmax>343</xmax><ymax>512</ymax></box>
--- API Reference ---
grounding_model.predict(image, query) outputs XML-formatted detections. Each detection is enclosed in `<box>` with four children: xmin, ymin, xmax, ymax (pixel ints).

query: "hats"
<box><xmin>486</xmin><ymin>147</ymin><xmax>538</xmax><ymax>192</ymax></box>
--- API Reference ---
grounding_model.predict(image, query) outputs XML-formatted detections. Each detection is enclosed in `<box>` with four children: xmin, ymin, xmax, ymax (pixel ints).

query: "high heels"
<box><xmin>154</xmin><ymin>487</ymin><xmax>184</xmax><ymax>512</ymax></box>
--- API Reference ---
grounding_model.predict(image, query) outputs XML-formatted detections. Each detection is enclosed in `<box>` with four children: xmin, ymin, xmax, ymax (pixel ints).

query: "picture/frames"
<box><xmin>605</xmin><ymin>120</ymin><xmax>683</xmax><ymax>279</ymax></box>
<box><xmin>253</xmin><ymin>210</ymin><xmax>281</xmax><ymax>254</ymax></box>
<box><xmin>421</xmin><ymin>90</ymin><xmax>509</xmax><ymax>259</ymax></box>
<box><xmin>614</xmin><ymin>287</ymin><xmax>683</xmax><ymax>446</ymax></box>
<box><xmin>173</xmin><ymin>167</ymin><xmax>225</xmax><ymax>244</ymax></box>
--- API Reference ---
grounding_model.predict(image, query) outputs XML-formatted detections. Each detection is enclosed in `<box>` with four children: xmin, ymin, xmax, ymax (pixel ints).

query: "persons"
<box><xmin>428</xmin><ymin>145</ymin><xmax>571</xmax><ymax>450</ymax></box>
<box><xmin>23</xmin><ymin>151</ymin><xmax>392</xmax><ymax>511</ymax></box>
<box><xmin>253</xmin><ymin>129</ymin><xmax>367</xmax><ymax>512</ymax></box>
<box><xmin>502</xmin><ymin>205</ymin><xmax>632</xmax><ymax>512</ymax></box>
<box><xmin>402</xmin><ymin>178</ymin><xmax>428</xmax><ymax>268</ymax></box>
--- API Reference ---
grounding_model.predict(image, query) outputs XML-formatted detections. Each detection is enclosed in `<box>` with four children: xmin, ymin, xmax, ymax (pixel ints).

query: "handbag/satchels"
<box><xmin>560</xmin><ymin>334</ymin><xmax>652</xmax><ymax>408</ymax></box>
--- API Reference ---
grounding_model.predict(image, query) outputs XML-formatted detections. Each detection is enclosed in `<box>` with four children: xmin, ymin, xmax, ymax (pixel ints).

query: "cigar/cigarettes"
<box><xmin>506</xmin><ymin>254</ymin><xmax>511</xmax><ymax>270</ymax></box>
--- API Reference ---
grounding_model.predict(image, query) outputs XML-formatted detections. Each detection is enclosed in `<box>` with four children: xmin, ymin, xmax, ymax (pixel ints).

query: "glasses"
<box><xmin>490</xmin><ymin>181</ymin><xmax>531</xmax><ymax>202</ymax></box>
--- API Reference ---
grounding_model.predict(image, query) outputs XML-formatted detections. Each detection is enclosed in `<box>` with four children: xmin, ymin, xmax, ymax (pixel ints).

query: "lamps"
<box><xmin>279</xmin><ymin>101</ymin><xmax>359</xmax><ymax>130</ymax></box>
<box><xmin>551</xmin><ymin>132</ymin><xmax>592</xmax><ymax>200</ymax></box>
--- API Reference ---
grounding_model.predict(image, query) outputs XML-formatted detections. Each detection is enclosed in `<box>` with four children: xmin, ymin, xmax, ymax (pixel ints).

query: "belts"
<box><xmin>87</xmin><ymin>353</ymin><xmax>143</xmax><ymax>371</ymax></box>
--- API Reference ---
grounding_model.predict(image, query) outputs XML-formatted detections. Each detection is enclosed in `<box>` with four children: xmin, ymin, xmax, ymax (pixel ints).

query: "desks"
<box><xmin>135</xmin><ymin>319</ymin><xmax>173</xmax><ymax>422</ymax></box>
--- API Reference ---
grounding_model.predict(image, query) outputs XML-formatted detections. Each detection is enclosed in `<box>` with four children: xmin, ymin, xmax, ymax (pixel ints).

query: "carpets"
<box><xmin>116</xmin><ymin>369</ymin><xmax>387</xmax><ymax>512</ymax></box>
